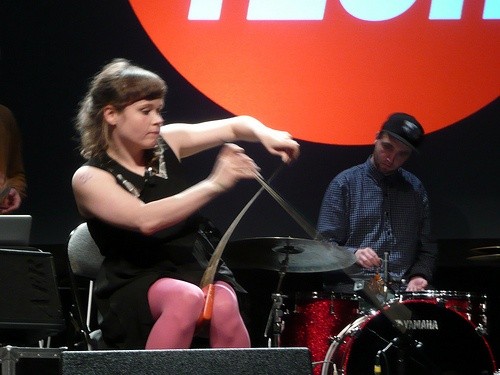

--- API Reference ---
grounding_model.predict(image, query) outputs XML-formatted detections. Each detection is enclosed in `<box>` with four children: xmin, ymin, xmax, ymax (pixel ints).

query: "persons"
<box><xmin>314</xmin><ymin>112</ymin><xmax>436</xmax><ymax>292</ymax></box>
<box><xmin>0</xmin><ymin>104</ymin><xmax>26</xmax><ymax>213</ymax></box>
<box><xmin>72</xmin><ymin>59</ymin><xmax>299</xmax><ymax>350</ymax></box>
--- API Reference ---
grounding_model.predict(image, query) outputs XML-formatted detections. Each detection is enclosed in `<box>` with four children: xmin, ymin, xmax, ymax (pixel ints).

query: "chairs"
<box><xmin>68</xmin><ymin>221</ymin><xmax>107</xmax><ymax>352</ymax></box>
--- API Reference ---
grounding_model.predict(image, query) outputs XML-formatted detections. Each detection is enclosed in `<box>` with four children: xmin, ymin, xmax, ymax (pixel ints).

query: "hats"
<box><xmin>381</xmin><ymin>113</ymin><xmax>424</xmax><ymax>155</ymax></box>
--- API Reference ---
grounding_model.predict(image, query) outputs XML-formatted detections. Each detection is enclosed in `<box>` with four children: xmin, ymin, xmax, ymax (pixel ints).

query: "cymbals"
<box><xmin>214</xmin><ymin>236</ymin><xmax>357</xmax><ymax>273</ymax></box>
<box><xmin>464</xmin><ymin>245</ymin><xmax>500</xmax><ymax>260</ymax></box>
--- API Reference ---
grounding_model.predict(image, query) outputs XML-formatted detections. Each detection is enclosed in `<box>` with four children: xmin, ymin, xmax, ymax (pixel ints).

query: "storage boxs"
<box><xmin>0</xmin><ymin>344</ymin><xmax>68</xmax><ymax>375</ymax></box>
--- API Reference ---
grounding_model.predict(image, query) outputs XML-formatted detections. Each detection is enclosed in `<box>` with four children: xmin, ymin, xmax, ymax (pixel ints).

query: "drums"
<box><xmin>397</xmin><ymin>289</ymin><xmax>477</xmax><ymax>330</ymax></box>
<box><xmin>319</xmin><ymin>298</ymin><xmax>499</xmax><ymax>375</ymax></box>
<box><xmin>261</xmin><ymin>290</ymin><xmax>358</xmax><ymax>375</ymax></box>
